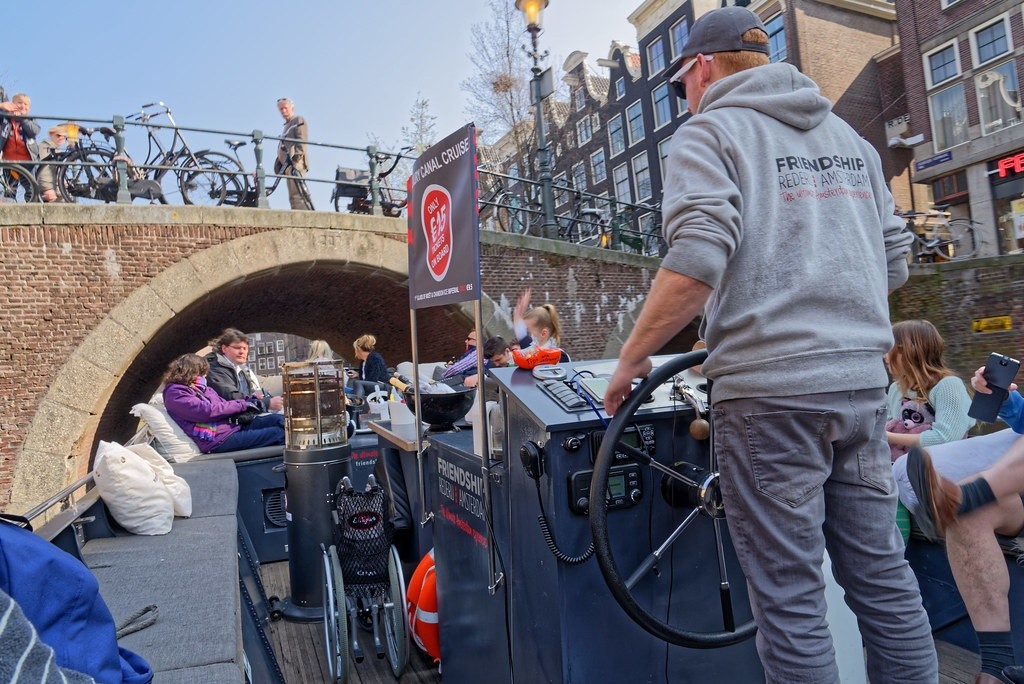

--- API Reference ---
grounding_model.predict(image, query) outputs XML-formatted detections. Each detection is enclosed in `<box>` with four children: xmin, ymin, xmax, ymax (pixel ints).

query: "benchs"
<box><xmin>1</xmin><ymin>424</ymin><xmax>286</xmax><ymax>684</ymax></box>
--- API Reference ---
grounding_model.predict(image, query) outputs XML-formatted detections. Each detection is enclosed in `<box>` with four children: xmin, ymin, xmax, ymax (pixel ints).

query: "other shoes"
<box><xmin>346</xmin><ymin>411</ymin><xmax>356</xmax><ymax>440</ymax></box>
<box><xmin>1001</xmin><ymin>666</ymin><xmax>1024</xmax><ymax>684</ymax></box>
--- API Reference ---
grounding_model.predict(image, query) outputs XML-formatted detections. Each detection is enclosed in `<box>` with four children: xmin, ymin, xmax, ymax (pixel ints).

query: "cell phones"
<box><xmin>982</xmin><ymin>352</ymin><xmax>1020</xmax><ymax>391</ymax></box>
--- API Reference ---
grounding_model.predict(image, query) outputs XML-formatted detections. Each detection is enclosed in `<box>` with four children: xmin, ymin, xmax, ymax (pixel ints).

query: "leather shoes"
<box><xmin>907</xmin><ymin>447</ymin><xmax>964</xmax><ymax>542</ymax></box>
<box><xmin>972</xmin><ymin>671</ymin><xmax>1006</xmax><ymax>684</ymax></box>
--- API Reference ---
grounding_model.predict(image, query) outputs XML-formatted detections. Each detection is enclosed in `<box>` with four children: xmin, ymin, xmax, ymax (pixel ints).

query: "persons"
<box><xmin>303</xmin><ymin>334</ymin><xmax>390</xmax><ymax>407</ymax></box>
<box><xmin>0</xmin><ymin>94</ymin><xmax>41</xmax><ymax>203</ymax></box>
<box><xmin>445</xmin><ymin>288</ymin><xmax>570</xmax><ymax>392</ymax></box>
<box><xmin>162</xmin><ymin>354</ymin><xmax>355</xmax><ymax>453</ymax></box>
<box><xmin>905</xmin><ymin>367</ymin><xmax>1024</xmax><ymax>684</ymax></box>
<box><xmin>882</xmin><ymin>319</ymin><xmax>976</xmax><ymax>550</ymax></box>
<box><xmin>35</xmin><ymin>126</ymin><xmax>73</xmax><ymax>204</ymax></box>
<box><xmin>602</xmin><ymin>6</ymin><xmax>940</xmax><ymax>684</ymax></box>
<box><xmin>274</xmin><ymin>98</ymin><xmax>311</xmax><ymax>210</ymax></box>
<box><xmin>205</xmin><ymin>328</ymin><xmax>284</xmax><ymax>414</ymax></box>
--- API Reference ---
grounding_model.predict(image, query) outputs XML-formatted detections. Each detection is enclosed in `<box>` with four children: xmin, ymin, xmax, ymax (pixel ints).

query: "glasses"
<box><xmin>670</xmin><ymin>54</ymin><xmax>714</xmax><ymax>100</ymax></box>
<box><xmin>465</xmin><ymin>337</ymin><xmax>476</xmax><ymax>341</ymax></box>
<box><xmin>228</xmin><ymin>345</ymin><xmax>248</xmax><ymax>350</ymax></box>
<box><xmin>277</xmin><ymin>98</ymin><xmax>287</xmax><ymax>102</ymax></box>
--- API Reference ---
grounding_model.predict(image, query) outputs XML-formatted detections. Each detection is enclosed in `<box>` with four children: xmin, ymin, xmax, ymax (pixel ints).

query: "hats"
<box><xmin>660</xmin><ymin>6</ymin><xmax>770</xmax><ymax>78</ymax></box>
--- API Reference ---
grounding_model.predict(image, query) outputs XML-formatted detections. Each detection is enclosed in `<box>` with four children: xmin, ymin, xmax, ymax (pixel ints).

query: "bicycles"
<box><xmin>330</xmin><ymin>145</ymin><xmax>416</xmax><ymax>217</ymax></box>
<box><xmin>605</xmin><ymin>199</ymin><xmax>669</xmax><ymax>258</ymax></box>
<box><xmin>893</xmin><ymin>204</ymin><xmax>984</xmax><ymax>261</ymax></box>
<box><xmin>511</xmin><ymin>178</ymin><xmax>604</xmax><ymax>248</ymax></box>
<box><xmin>477</xmin><ymin>153</ymin><xmax>530</xmax><ymax>236</ymax></box>
<box><xmin>180</xmin><ymin>123</ymin><xmax>315</xmax><ymax>211</ymax></box>
<box><xmin>0</xmin><ymin>164</ymin><xmax>38</xmax><ymax>204</ymax></box>
<box><xmin>34</xmin><ymin>101</ymin><xmax>247</xmax><ymax>206</ymax></box>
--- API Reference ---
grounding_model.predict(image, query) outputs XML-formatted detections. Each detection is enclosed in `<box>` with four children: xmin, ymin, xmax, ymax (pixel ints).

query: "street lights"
<box><xmin>515</xmin><ymin>0</ymin><xmax>559</xmax><ymax>241</ymax></box>
<box><xmin>887</xmin><ymin>138</ymin><xmax>916</xmax><ymax>218</ymax></box>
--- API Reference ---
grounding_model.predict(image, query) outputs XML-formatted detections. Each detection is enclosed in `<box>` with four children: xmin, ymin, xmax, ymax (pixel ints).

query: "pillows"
<box><xmin>147</xmin><ymin>393</ymin><xmax>163</xmax><ymax>404</ymax></box>
<box><xmin>129</xmin><ymin>403</ymin><xmax>202</xmax><ymax>463</ymax></box>
<box><xmin>93</xmin><ymin>440</ymin><xmax>174</xmax><ymax>535</ymax></box>
<box><xmin>124</xmin><ymin>442</ymin><xmax>192</xmax><ymax>517</ymax></box>
